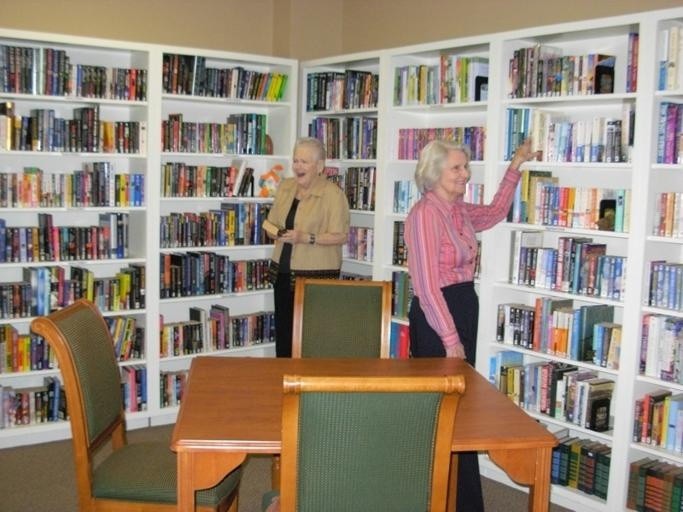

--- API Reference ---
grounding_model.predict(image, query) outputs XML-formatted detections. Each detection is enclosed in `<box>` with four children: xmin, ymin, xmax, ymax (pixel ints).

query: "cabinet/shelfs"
<box><xmin>300</xmin><ymin>7</ymin><xmax>683</xmax><ymax>512</ymax></box>
<box><xmin>0</xmin><ymin>27</ymin><xmax>299</xmax><ymax>448</ymax></box>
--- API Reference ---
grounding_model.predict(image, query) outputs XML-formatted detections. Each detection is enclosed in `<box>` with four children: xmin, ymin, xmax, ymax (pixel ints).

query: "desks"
<box><xmin>167</xmin><ymin>351</ymin><xmax>559</xmax><ymax>512</ymax></box>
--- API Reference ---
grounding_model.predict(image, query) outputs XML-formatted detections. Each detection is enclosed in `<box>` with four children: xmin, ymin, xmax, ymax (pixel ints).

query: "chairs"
<box><xmin>259</xmin><ymin>374</ymin><xmax>465</xmax><ymax>512</ymax></box>
<box><xmin>29</xmin><ymin>296</ymin><xmax>242</xmax><ymax>512</ymax></box>
<box><xmin>269</xmin><ymin>275</ymin><xmax>392</xmax><ymax>492</ymax></box>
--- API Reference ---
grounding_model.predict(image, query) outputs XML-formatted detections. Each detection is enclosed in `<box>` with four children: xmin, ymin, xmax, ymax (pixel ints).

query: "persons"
<box><xmin>259</xmin><ymin>135</ymin><xmax>349</xmax><ymax>358</ymax></box>
<box><xmin>404</xmin><ymin>135</ymin><xmax>540</xmax><ymax>371</ymax></box>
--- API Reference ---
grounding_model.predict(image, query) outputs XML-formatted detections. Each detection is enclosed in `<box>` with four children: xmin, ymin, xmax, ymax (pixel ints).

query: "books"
<box><xmin>306</xmin><ymin>69</ymin><xmax>376</xmax><ymax>285</ymax></box>
<box><xmin>386</xmin><ymin>54</ymin><xmax>489</xmax><ymax>360</ymax></box>
<box><xmin>0</xmin><ymin>42</ymin><xmax>149</xmax><ymax>428</ymax></box>
<box><xmin>489</xmin><ymin>34</ymin><xmax>614</xmax><ymax>502</ymax></box>
<box><xmin>156</xmin><ymin>54</ymin><xmax>287</xmax><ymax>407</ymax></box>
<box><xmin>624</xmin><ymin>32</ymin><xmax>683</xmax><ymax>512</ymax></box>
